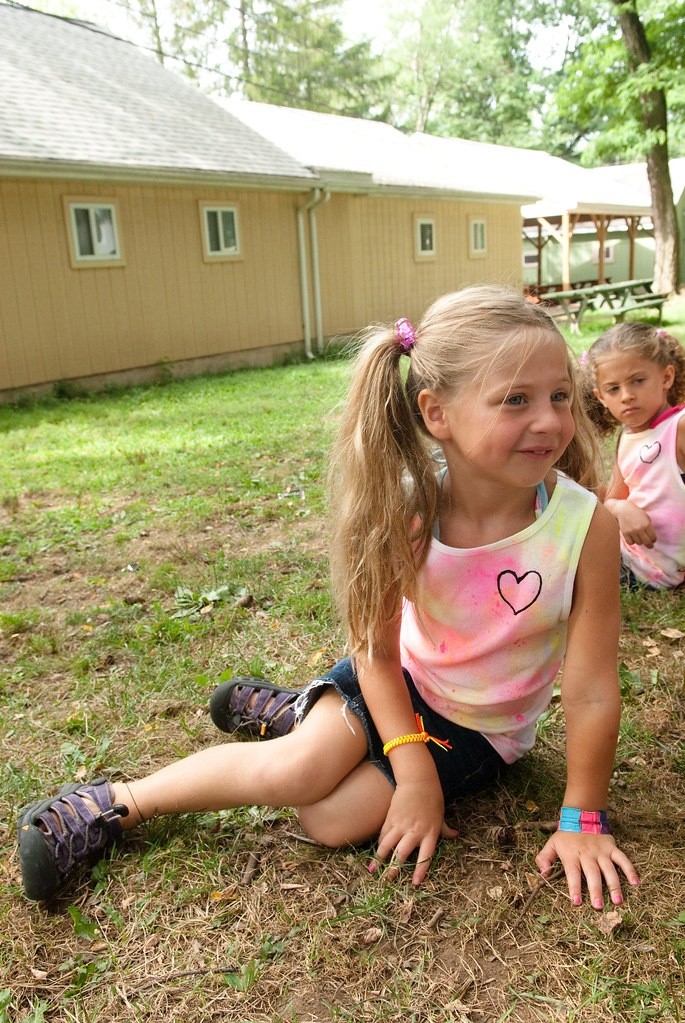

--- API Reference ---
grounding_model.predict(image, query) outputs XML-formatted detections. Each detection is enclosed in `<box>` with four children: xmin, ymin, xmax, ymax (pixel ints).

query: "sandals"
<box><xmin>209</xmin><ymin>680</ymin><xmax>303</xmax><ymax>740</ymax></box>
<box><xmin>20</xmin><ymin>778</ymin><xmax>130</xmax><ymax>900</ymax></box>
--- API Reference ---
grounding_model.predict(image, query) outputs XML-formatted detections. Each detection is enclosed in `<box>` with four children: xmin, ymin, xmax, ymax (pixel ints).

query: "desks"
<box><xmin>538</xmin><ymin>278</ymin><xmax>654</xmax><ymax>334</ymax></box>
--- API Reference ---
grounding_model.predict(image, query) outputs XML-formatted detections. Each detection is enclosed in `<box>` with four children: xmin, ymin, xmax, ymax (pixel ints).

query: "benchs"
<box><xmin>549</xmin><ymin>305</ymin><xmax>592</xmax><ymax>317</ymax></box>
<box><xmin>610</xmin><ymin>297</ymin><xmax>668</xmax><ymax>315</ymax></box>
<box><xmin>632</xmin><ymin>291</ymin><xmax>671</xmax><ymax>302</ymax></box>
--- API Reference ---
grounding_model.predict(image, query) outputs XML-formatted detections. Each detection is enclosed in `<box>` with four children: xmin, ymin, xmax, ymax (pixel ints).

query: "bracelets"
<box><xmin>558</xmin><ymin>807</ymin><xmax>612</xmax><ymax>834</ymax></box>
<box><xmin>383</xmin><ymin>713</ymin><xmax>453</xmax><ymax>756</ymax></box>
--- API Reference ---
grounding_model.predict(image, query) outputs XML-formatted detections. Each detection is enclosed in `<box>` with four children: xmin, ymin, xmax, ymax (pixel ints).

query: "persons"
<box><xmin>21</xmin><ymin>285</ymin><xmax>639</xmax><ymax>908</ymax></box>
<box><xmin>579</xmin><ymin>323</ymin><xmax>685</xmax><ymax>588</ymax></box>
